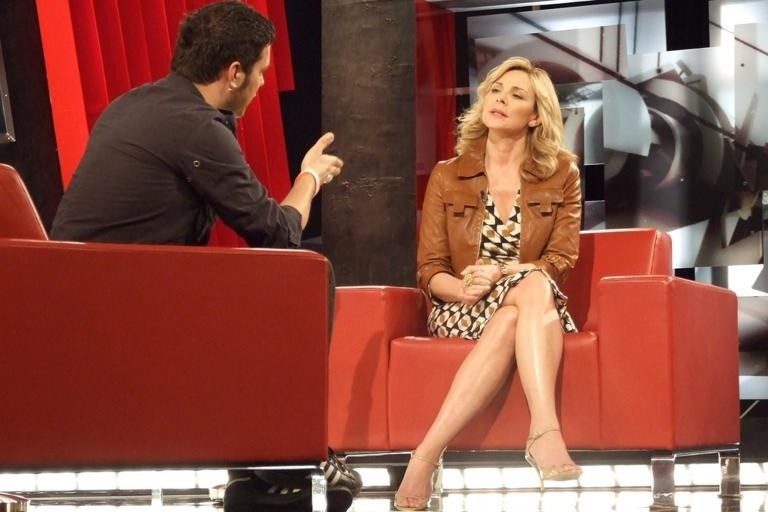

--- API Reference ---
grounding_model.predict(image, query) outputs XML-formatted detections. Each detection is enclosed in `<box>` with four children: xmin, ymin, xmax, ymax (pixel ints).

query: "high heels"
<box><xmin>524</xmin><ymin>425</ymin><xmax>582</xmax><ymax>491</ymax></box>
<box><xmin>394</xmin><ymin>444</ymin><xmax>441</xmax><ymax>511</ymax></box>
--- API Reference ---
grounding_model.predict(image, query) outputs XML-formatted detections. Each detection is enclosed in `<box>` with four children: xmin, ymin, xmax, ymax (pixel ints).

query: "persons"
<box><xmin>50</xmin><ymin>1</ymin><xmax>362</xmax><ymax>512</ymax></box>
<box><xmin>392</xmin><ymin>56</ymin><xmax>583</xmax><ymax>510</ymax></box>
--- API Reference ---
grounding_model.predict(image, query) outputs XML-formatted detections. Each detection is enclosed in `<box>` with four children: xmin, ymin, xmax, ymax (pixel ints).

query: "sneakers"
<box><xmin>252</xmin><ymin>447</ymin><xmax>361</xmax><ymax>495</ymax></box>
<box><xmin>224</xmin><ymin>475</ymin><xmax>352</xmax><ymax>512</ymax></box>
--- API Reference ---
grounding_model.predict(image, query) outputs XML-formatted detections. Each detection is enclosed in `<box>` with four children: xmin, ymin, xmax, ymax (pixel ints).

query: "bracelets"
<box><xmin>294</xmin><ymin>167</ymin><xmax>321</xmax><ymax>198</ymax></box>
<box><xmin>500</xmin><ymin>265</ymin><xmax>509</xmax><ymax>278</ymax></box>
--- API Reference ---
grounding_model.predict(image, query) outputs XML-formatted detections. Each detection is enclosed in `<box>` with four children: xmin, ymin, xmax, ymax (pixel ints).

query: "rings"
<box><xmin>464</xmin><ymin>274</ymin><xmax>472</xmax><ymax>286</ymax></box>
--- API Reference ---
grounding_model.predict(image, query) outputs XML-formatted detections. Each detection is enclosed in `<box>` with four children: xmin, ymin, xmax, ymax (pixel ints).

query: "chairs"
<box><xmin>330</xmin><ymin>227</ymin><xmax>742</xmax><ymax>512</ymax></box>
<box><xmin>0</xmin><ymin>161</ymin><xmax>333</xmax><ymax>512</ymax></box>
